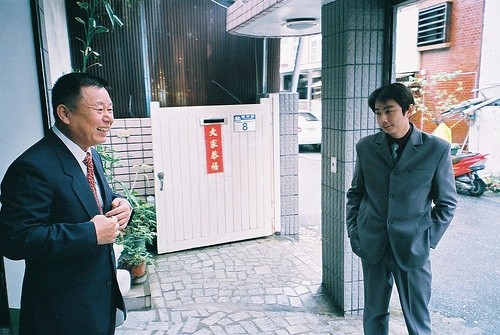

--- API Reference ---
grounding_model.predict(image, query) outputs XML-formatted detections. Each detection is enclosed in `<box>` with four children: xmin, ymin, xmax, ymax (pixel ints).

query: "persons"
<box><xmin>346</xmin><ymin>83</ymin><xmax>458</xmax><ymax>335</ymax></box>
<box><xmin>429</xmin><ymin>116</ymin><xmax>453</xmax><ymax>145</ymax></box>
<box><xmin>0</xmin><ymin>73</ymin><xmax>135</xmax><ymax>335</ymax></box>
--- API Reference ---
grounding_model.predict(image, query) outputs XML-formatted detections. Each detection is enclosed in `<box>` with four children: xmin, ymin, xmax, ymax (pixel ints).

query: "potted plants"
<box><xmin>119</xmin><ymin>200</ymin><xmax>157</xmax><ymax>252</ymax></box>
<box><xmin>117</xmin><ymin>245</ymin><xmax>156</xmax><ymax>282</ymax></box>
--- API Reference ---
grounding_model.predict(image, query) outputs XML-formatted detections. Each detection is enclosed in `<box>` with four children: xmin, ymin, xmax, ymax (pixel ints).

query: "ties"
<box><xmin>82</xmin><ymin>152</ymin><xmax>103</xmax><ymax>216</ymax></box>
<box><xmin>391</xmin><ymin>142</ymin><xmax>399</xmax><ymax>163</ymax></box>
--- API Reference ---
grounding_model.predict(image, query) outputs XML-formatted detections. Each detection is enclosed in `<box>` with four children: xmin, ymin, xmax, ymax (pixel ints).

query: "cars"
<box><xmin>297</xmin><ymin>111</ymin><xmax>322</xmax><ymax>150</ymax></box>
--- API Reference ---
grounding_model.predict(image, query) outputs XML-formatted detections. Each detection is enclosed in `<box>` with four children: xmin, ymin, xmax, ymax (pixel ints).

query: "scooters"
<box><xmin>452</xmin><ymin>150</ymin><xmax>488</xmax><ymax>197</ymax></box>
